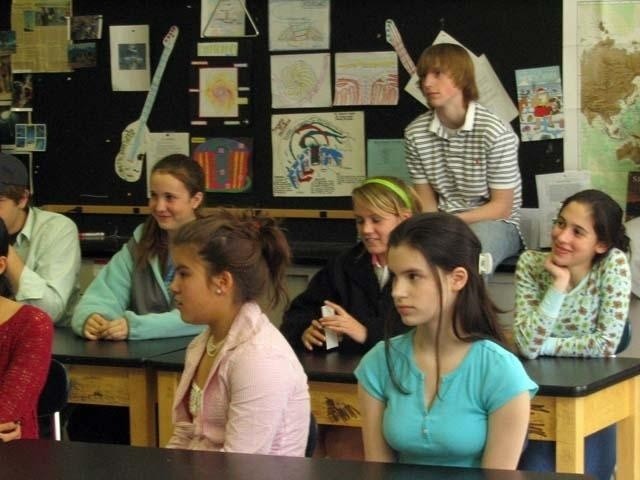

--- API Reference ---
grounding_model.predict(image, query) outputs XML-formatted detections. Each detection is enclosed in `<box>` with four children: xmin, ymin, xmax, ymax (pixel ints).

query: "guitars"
<box><xmin>114</xmin><ymin>25</ymin><xmax>180</xmax><ymax>183</ymax></box>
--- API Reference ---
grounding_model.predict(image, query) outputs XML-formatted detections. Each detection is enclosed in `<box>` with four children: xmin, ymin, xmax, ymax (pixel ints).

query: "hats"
<box><xmin>0</xmin><ymin>153</ymin><xmax>28</xmax><ymax>186</ymax></box>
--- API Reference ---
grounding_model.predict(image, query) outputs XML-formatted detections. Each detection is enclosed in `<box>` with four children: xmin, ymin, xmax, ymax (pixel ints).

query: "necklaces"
<box><xmin>205</xmin><ymin>335</ymin><xmax>228</xmax><ymax>357</ymax></box>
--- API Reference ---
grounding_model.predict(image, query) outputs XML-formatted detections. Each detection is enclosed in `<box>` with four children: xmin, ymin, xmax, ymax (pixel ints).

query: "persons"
<box><xmin>2</xmin><ymin>152</ymin><xmax>83</xmax><ymax>324</ymax></box>
<box><xmin>512</xmin><ymin>189</ymin><xmax>632</xmax><ymax>480</ymax></box>
<box><xmin>276</xmin><ymin>176</ymin><xmax>424</xmax><ymax>357</ymax></box>
<box><xmin>70</xmin><ymin>153</ymin><xmax>213</xmax><ymax>341</ymax></box>
<box><xmin>1</xmin><ymin>222</ymin><xmax>58</xmax><ymax>445</ymax></box>
<box><xmin>161</xmin><ymin>202</ymin><xmax>314</xmax><ymax>459</ymax></box>
<box><xmin>402</xmin><ymin>42</ymin><xmax>524</xmax><ymax>291</ymax></box>
<box><xmin>351</xmin><ymin>212</ymin><xmax>540</xmax><ymax>472</ymax></box>
